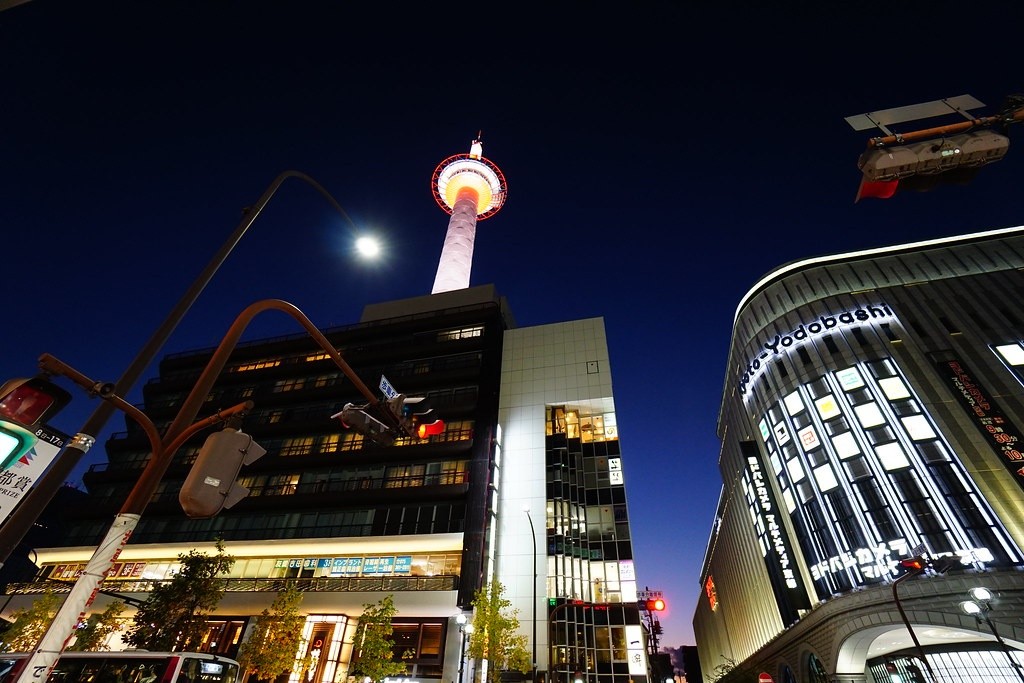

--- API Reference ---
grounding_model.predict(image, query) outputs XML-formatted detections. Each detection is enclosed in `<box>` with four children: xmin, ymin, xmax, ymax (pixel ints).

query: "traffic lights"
<box><xmin>901</xmin><ymin>555</ymin><xmax>926</xmax><ymax>573</ymax></box>
<box><xmin>387</xmin><ymin>394</ymin><xmax>444</xmax><ymax>442</ymax></box>
<box><xmin>179</xmin><ymin>428</ymin><xmax>267</xmax><ymax>519</ymax></box>
<box><xmin>0</xmin><ymin>378</ymin><xmax>74</xmax><ymax>473</ymax></box>
<box><xmin>638</xmin><ymin>600</ymin><xmax>665</xmax><ymax>610</ymax></box>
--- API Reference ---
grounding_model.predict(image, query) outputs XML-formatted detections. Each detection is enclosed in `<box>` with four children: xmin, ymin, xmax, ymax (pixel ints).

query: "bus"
<box><xmin>0</xmin><ymin>649</ymin><xmax>240</xmax><ymax>683</ymax></box>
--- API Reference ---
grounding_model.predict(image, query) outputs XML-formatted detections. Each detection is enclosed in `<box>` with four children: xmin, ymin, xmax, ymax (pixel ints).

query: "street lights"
<box><xmin>959</xmin><ymin>587</ymin><xmax>1024</xmax><ymax>683</ymax></box>
<box><xmin>0</xmin><ymin>170</ymin><xmax>383</xmax><ymax>570</ymax></box>
<box><xmin>522</xmin><ymin>505</ymin><xmax>537</xmax><ymax>683</ymax></box>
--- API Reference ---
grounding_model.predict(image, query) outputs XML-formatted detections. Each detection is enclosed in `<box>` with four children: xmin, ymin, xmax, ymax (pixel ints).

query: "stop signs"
<box><xmin>759</xmin><ymin>673</ymin><xmax>773</xmax><ymax>683</ymax></box>
